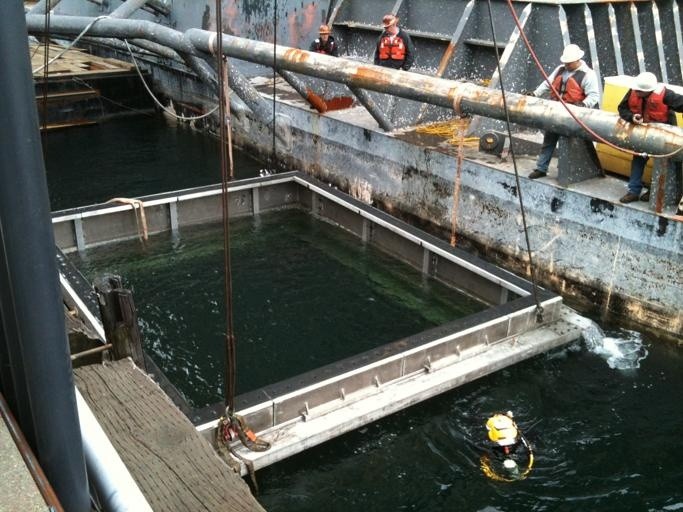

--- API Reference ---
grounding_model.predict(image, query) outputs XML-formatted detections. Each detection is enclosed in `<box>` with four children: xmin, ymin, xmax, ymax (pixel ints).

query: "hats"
<box><xmin>317</xmin><ymin>25</ymin><xmax>333</xmax><ymax>34</ymax></box>
<box><xmin>631</xmin><ymin>72</ymin><xmax>657</xmax><ymax>92</ymax></box>
<box><xmin>559</xmin><ymin>44</ymin><xmax>584</xmax><ymax>63</ymax></box>
<box><xmin>381</xmin><ymin>14</ymin><xmax>399</xmax><ymax>28</ymax></box>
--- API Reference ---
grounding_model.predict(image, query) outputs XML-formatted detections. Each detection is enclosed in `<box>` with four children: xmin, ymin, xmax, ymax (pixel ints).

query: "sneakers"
<box><xmin>528</xmin><ymin>169</ymin><xmax>546</xmax><ymax>178</ymax></box>
<box><xmin>619</xmin><ymin>191</ymin><xmax>650</xmax><ymax>203</ymax></box>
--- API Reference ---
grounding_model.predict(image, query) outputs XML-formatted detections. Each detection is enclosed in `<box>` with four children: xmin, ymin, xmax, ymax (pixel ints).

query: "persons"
<box><xmin>310</xmin><ymin>24</ymin><xmax>338</xmax><ymax>57</ymax></box>
<box><xmin>479</xmin><ymin>409</ymin><xmax>540</xmax><ymax>485</ymax></box>
<box><xmin>618</xmin><ymin>71</ymin><xmax>683</xmax><ymax>204</ymax></box>
<box><xmin>374</xmin><ymin>13</ymin><xmax>416</xmax><ymax>71</ymax></box>
<box><xmin>521</xmin><ymin>43</ymin><xmax>601</xmax><ymax>179</ymax></box>
